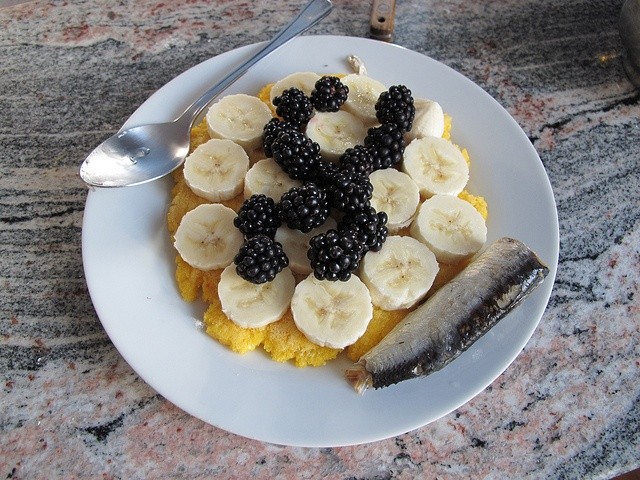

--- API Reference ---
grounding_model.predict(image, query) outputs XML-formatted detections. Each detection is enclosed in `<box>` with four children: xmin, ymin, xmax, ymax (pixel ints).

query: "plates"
<box><xmin>81</xmin><ymin>34</ymin><xmax>560</xmax><ymax>448</ymax></box>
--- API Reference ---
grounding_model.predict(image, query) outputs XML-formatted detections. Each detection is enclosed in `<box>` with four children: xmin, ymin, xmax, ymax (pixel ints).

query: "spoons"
<box><xmin>79</xmin><ymin>1</ymin><xmax>334</xmax><ymax>190</ymax></box>
